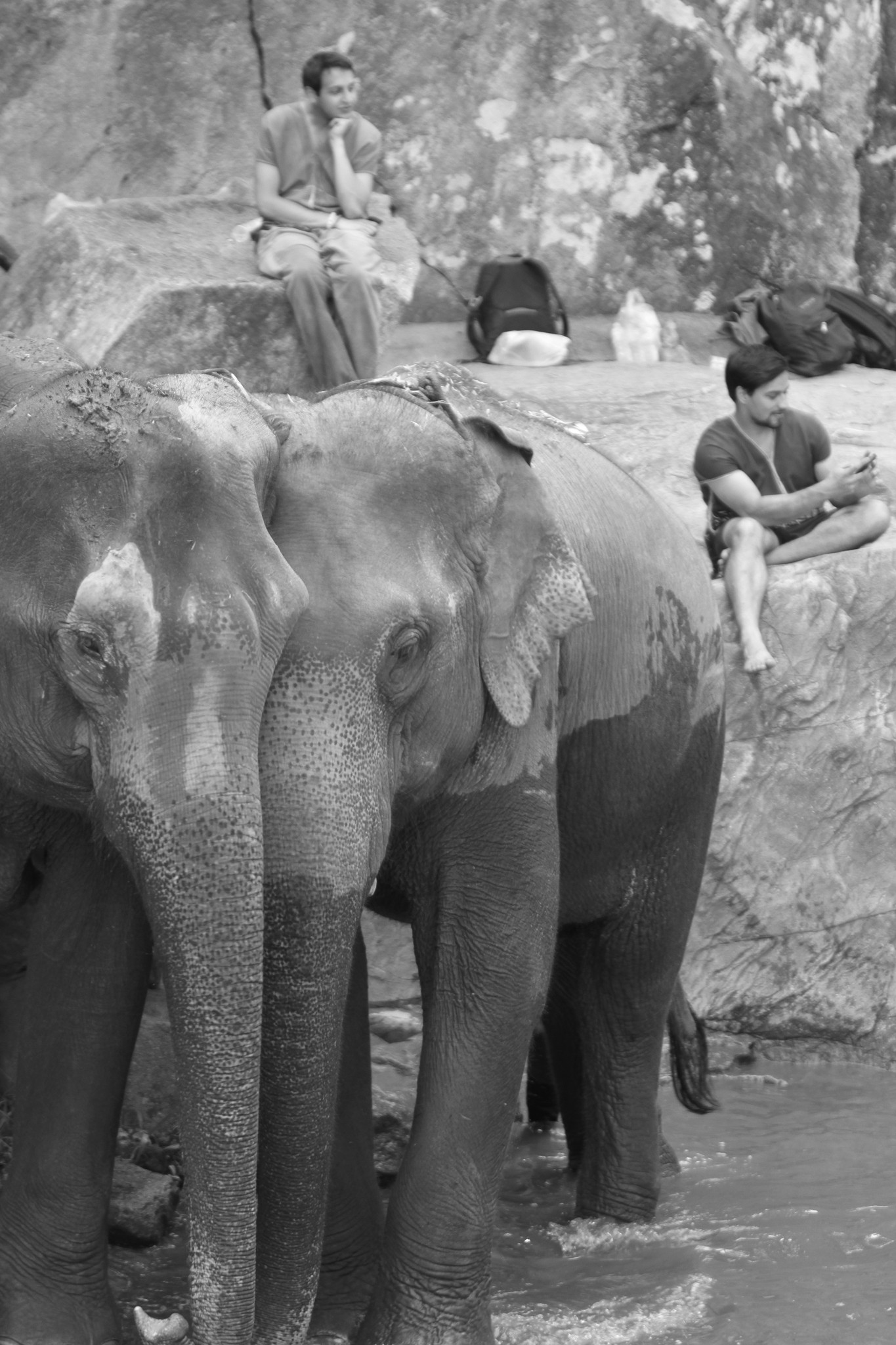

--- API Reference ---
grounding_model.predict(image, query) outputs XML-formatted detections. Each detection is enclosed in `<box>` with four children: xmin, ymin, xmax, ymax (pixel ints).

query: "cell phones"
<box><xmin>856</xmin><ymin>453</ymin><xmax>876</xmax><ymax>473</ymax></box>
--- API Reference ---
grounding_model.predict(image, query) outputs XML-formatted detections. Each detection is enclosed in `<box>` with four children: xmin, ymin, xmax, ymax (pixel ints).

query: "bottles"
<box><xmin>233</xmin><ymin>217</ymin><xmax>264</xmax><ymax>243</ymax></box>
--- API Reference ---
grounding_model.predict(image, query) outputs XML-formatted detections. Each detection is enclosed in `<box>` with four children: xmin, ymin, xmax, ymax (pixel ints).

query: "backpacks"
<box><xmin>465</xmin><ymin>253</ymin><xmax>569</xmax><ymax>363</ymax></box>
<box><xmin>823</xmin><ymin>284</ymin><xmax>896</xmax><ymax>370</ymax></box>
<box><xmin>758</xmin><ymin>280</ymin><xmax>855</xmax><ymax>377</ymax></box>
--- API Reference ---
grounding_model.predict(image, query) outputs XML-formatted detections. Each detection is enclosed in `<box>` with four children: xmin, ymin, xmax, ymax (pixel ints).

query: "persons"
<box><xmin>254</xmin><ymin>52</ymin><xmax>382</xmax><ymax>392</ymax></box>
<box><xmin>694</xmin><ymin>343</ymin><xmax>890</xmax><ymax>674</ymax></box>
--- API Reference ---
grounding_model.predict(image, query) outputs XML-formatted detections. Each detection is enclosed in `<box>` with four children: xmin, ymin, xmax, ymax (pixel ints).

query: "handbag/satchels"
<box><xmin>611</xmin><ymin>288</ymin><xmax>661</xmax><ymax>364</ymax></box>
<box><xmin>488</xmin><ymin>330</ymin><xmax>572</xmax><ymax>366</ymax></box>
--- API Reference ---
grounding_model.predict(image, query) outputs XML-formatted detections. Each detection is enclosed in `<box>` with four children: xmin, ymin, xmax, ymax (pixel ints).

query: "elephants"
<box><xmin>0</xmin><ymin>367</ymin><xmax>728</xmax><ymax>1345</ymax></box>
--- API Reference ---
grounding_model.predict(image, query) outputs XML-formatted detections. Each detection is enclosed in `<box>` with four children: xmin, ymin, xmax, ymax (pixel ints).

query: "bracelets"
<box><xmin>327</xmin><ymin>212</ymin><xmax>337</xmax><ymax>228</ymax></box>
